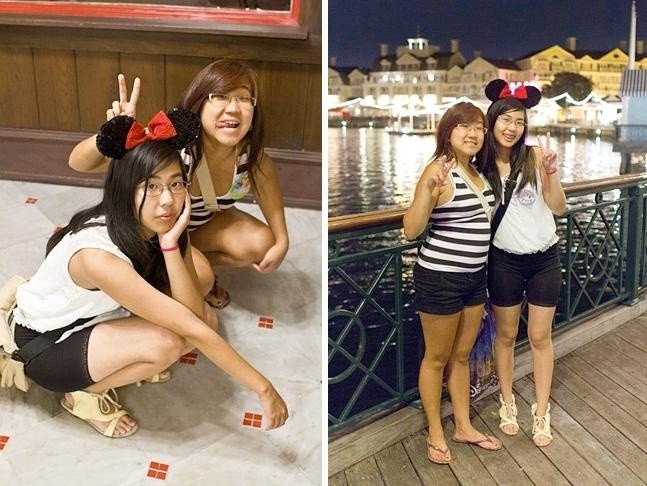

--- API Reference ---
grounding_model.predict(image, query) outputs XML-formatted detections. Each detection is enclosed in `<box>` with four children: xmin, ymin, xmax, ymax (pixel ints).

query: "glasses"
<box><xmin>208</xmin><ymin>92</ymin><xmax>257</xmax><ymax>110</ymax></box>
<box><xmin>456</xmin><ymin>123</ymin><xmax>488</xmax><ymax>135</ymax></box>
<box><xmin>135</xmin><ymin>180</ymin><xmax>191</xmax><ymax>198</ymax></box>
<box><xmin>499</xmin><ymin>114</ymin><xmax>526</xmax><ymax>128</ymax></box>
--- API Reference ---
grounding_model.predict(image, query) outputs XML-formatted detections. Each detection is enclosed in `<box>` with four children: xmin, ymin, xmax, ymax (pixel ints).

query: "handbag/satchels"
<box><xmin>0</xmin><ymin>293</ymin><xmax>33</xmax><ymax>393</ymax></box>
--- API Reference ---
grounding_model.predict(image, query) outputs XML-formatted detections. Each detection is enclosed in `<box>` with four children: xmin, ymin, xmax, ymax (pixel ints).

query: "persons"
<box><xmin>67</xmin><ymin>56</ymin><xmax>287</xmax><ymax>308</ymax></box>
<box><xmin>473</xmin><ymin>78</ymin><xmax>571</xmax><ymax>448</ymax></box>
<box><xmin>402</xmin><ymin>101</ymin><xmax>503</xmax><ymax>464</ymax></box>
<box><xmin>12</xmin><ymin>107</ymin><xmax>288</xmax><ymax>440</ymax></box>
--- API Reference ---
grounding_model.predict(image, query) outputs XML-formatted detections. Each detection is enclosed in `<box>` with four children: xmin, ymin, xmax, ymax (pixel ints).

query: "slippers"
<box><xmin>453</xmin><ymin>433</ymin><xmax>503</xmax><ymax>451</ymax></box>
<box><xmin>204</xmin><ymin>285</ymin><xmax>232</xmax><ymax>311</ymax></box>
<box><xmin>426</xmin><ymin>437</ymin><xmax>451</xmax><ymax>464</ymax></box>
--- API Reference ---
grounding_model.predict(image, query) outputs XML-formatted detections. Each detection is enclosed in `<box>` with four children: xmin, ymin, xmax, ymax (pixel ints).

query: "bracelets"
<box><xmin>158</xmin><ymin>244</ymin><xmax>179</xmax><ymax>252</ymax></box>
<box><xmin>546</xmin><ymin>169</ymin><xmax>557</xmax><ymax>175</ymax></box>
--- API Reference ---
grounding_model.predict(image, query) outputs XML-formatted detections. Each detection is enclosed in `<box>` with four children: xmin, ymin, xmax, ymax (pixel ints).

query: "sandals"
<box><xmin>146</xmin><ymin>370</ymin><xmax>171</xmax><ymax>383</ymax></box>
<box><xmin>61</xmin><ymin>388</ymin><xmax>139</xmax><ymax>438</ymax></box>
<box><xmin>498</xmin><ymin>393</ymin><xmax>520</xmax><ymax>435</ymax></box>
<box><xmin>531</xmin><ymin>402</ymin><xmax>553</xmax><ymax>447</ymax></box>
